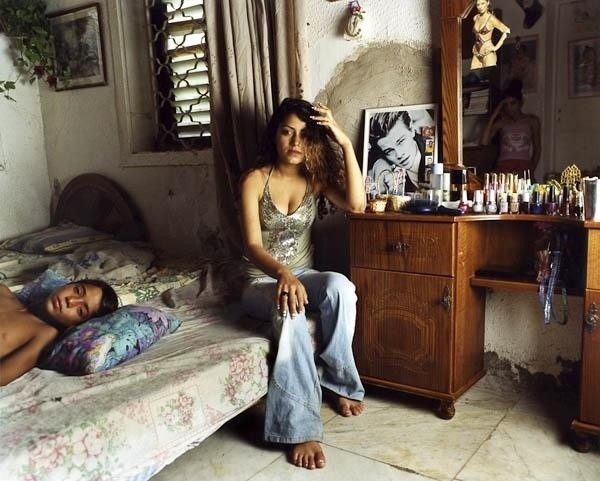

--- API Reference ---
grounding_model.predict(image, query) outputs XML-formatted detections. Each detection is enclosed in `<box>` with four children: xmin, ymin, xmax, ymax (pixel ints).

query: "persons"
<box><xmin>53</xmin><ymin>21</ymin><xmax>98</xmax><ymax>79</ymax></box>
<box><xmin>457</xmin><ymin>0</ymin><xmax>543</xmax><ymax>188</ymax></box>
<box><xmin>0</xmin><ymin>277</ymin><xmax>119</xmax><ymax>389</ymax></box>
<box><xmin>365</xmin><ymin>106</ymin><xmax>436</xmax><ymax>202</ymax></box>
<box><xmin>222</xmin><ymin>97</ymin><xmax>370</xmax><ymax>472</ymax></box>
<box><xmin>576</xmin><ymin>44</ymin><xmax>600</xmax><ymax>94</ymax></box>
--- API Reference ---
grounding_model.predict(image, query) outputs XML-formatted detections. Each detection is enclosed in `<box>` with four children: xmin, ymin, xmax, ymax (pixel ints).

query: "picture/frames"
<box><xmin>568</xmin><ymin>36</ymin><xmax>600</xmax><ymax>98</ymax></box>
<box><xmin>499</xmin><ymin>33</ymin><xmax>540</xmax><ymax>98</ymax></box>
<box><xmin>45</xmin><ymin>3</ymin><xmax>108</xmax><ymax>92</ymax></box>
<box><xmin>362</xmin><ymin>103</ymin><xmax>439</xmax><ymax>199</ymax></box>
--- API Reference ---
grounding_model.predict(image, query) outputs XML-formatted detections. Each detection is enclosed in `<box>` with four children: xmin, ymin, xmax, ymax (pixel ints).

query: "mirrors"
<box><xmin>440</xmin><ymin>0</ymin><xmax>600</xmax><ymax>193</ymax></box>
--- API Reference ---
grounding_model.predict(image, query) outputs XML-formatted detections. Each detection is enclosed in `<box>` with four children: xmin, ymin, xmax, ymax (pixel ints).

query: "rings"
<box><xmin>281</xmin><ymin>291</ymin><xmax>289</xmax><ymax>297</ymax></box>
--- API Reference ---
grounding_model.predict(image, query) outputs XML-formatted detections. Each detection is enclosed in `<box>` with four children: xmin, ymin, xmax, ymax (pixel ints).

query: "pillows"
<box><xmin>0</xmin><ymin>219</ymin><xmax>113</xmax><ymax>254</ymax></box>
<box><xmin>17</xmin><ymin>259</ymin><xmax>183</xmax><ymax>377</ymax></box>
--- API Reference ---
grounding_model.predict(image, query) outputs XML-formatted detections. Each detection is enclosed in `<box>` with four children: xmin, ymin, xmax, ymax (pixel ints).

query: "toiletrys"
<box><xmin>426</xmin><ymin>162</ymin><xmax>586</xmax><ymax>221</ymax></box>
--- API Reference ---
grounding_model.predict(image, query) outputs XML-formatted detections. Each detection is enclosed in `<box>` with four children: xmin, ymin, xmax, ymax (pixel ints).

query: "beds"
<box><xmin>0</xmin><ymin>172</ymin><xmax>315</xmax><ymax>481</ymax></box>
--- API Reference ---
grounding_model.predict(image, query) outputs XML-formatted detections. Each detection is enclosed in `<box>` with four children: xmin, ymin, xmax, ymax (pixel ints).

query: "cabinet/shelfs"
<box><xmin>345</xmin><ymin>209</ymin><xmax>600</xmax><ymax>453</ymax></box>
